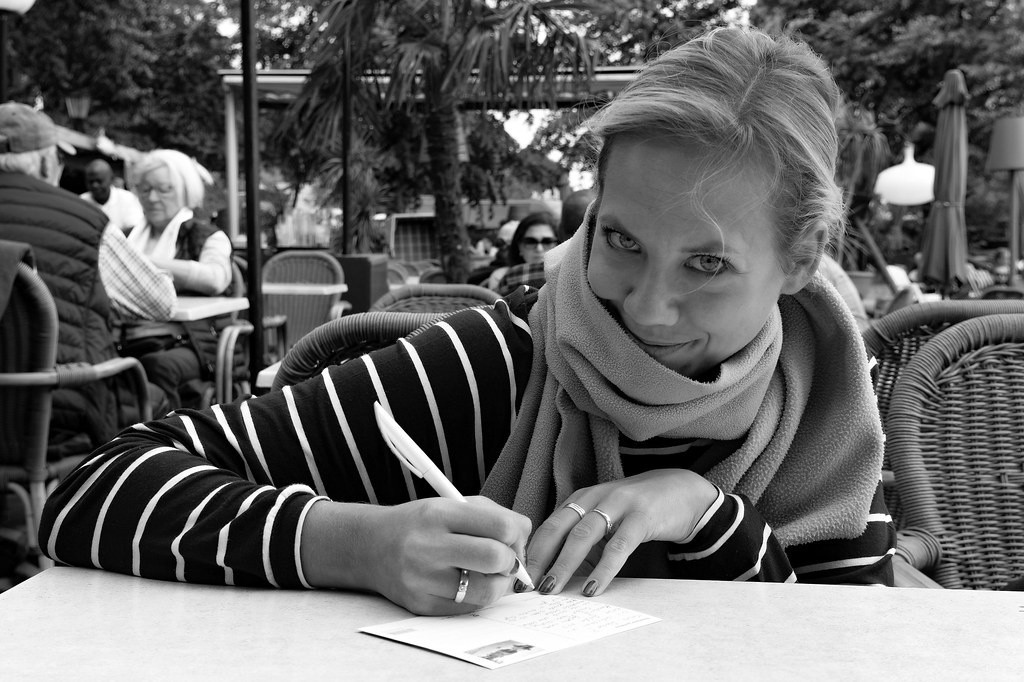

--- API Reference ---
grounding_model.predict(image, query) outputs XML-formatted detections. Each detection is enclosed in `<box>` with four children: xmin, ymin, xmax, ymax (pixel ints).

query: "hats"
<box><xmin>0</xmin><ymin>100</ymin><xmax>77</xmax><ymax>155</ymax></box>
<box><xmin>498</xmin><ymin>220</ymin><xmax>520</xmax><ymax>246</ymax></box>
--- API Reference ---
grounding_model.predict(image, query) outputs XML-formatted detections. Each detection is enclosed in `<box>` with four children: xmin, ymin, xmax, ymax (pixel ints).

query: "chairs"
<box><xmin>233</xmin><ymin>255</ymin><xmax>292</xmax><ymax>383</ymax></box>
<box><xmin>0</xmin><ymin>239</ymin><xmax>153</xmax><ymax>572</ymax></box>
<box><xmin>862</xmin><ymin>300</ymin><xmax>1024</xmax><ymax>532</ymax></box>
<box><xmin>884</xmin><ymin>311</ymin><xmax>1024</xmax><ymax>592</ymax></box>
<box><xmin>270</xmin><ymin>312</ymin><xmax>447</xmax><ymax>392</ymax></box>
<box><xmin>366</xmin><ymin>282</ymin><xmax>503</xmax><ymax>313</ymax></box>
<box><xmin>181</xmin><ymin>262</ymin><xmax>255</xmax><ymax>409</ymax></box>
<box><xmin>262</xmin><ymin>250</ymin><xmax>354</xmax><ymax>362</ymax></box>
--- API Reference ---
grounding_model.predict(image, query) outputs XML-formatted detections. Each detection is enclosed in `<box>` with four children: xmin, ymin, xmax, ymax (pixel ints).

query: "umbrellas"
<box><xmin>915</xmin><ymin>67</ymin><xmax>976</xmax><ymax>299</ymax></box>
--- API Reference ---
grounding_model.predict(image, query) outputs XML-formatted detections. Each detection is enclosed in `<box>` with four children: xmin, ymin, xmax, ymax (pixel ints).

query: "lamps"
<box><xmin>990</xmin><ymin>116</ymin><xmax>1024</xmax><ymax>287</ymax></box>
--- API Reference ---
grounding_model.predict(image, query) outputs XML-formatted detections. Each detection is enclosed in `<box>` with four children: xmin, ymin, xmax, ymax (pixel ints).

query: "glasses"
<box><xmin>522</xmin><ymin>238</ymin><xmax>558</xmax><ymax>249</ymax></box>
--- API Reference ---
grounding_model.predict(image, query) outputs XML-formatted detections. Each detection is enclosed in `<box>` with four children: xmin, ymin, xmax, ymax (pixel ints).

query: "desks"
<box><xmin>262</xmin><ymin>281</ymin><xmax>349</xmax><ymax>296</ymax></box>
<box><xmin>0</xmin><ymin>564</ymin><xmax>1024</xmax><ymax>682</ymax></box>
<box><xmin>112</xmin><ymin>295</ymin><xmax>249</xmax><ymax>341</ymax></box>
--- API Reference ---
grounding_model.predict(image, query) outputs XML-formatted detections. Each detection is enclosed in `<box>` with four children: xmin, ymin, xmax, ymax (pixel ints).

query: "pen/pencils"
<box><xmin>372</xmin><ymin>400</ymin><xmax>535</xmax><ymax>588</ymax></box>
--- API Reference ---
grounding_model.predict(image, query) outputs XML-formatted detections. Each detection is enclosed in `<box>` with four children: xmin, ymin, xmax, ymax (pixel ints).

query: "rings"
<box><xmin>588</xmin><ymin>508</ymin><xmax>612</xmax><ymax>535</ymax></box>
<box><xmin>455</xmin><ymin>569</ymin><xmax>469</xmax><ymax>602</ymax></box>
<box><xmin>562</xmin><ymin>502</ymin><xmax>585</xmax><ymax>519</ymax></box>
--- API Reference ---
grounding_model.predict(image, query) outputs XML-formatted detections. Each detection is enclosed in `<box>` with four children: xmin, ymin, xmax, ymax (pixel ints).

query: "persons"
<box><xmin>476</xmin><ymin>211</ymin><xmax>554</xmax><ymax>290</ymax></box>
<box><xmin>37</xmin><ymin>28</ymin><xmax>897</xmax><ymax>619</ymax></box>
<box><xmin>556</xmin><ymin>188</ymin><xmax>596</xmax><ymax>246</ymax></box>
<box><xmin>0</xmin><ymin>102</ymin><xmax>169</xmax><ymax>460</ymax></box>
<box><xmin>991</xmin><ymin>248</ymin><xmax>1013</xmax><ymax>276</ymax></box>
<box><xmin>112</xmin><ymin>149</ymin><xmax>248</xmax><ymax>425</ymax></box>
<box><xmin>74</xmin><ymin>160</ymin><xmax>145</xmax><ymax>236</ymax></box>
<box><xmin>466</xmin><ymin>218</ymin><xmax>525</xmax><ymax>286</ymax></box>
